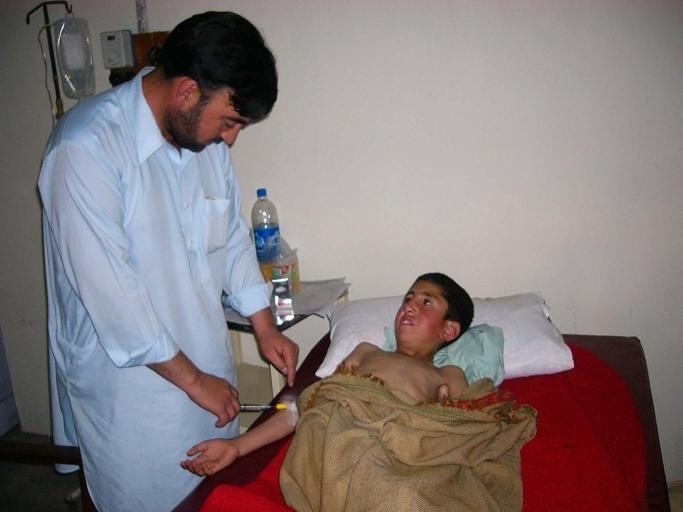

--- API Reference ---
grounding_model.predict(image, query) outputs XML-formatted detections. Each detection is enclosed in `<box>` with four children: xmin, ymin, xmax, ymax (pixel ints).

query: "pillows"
<box><xmin>313</xmin><ymin>290</ymin><xmax>576</xmax><ymax>386</ymax></box>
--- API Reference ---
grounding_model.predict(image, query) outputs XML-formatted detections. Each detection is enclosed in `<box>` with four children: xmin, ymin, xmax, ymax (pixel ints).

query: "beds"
<box><xmin>169</xmin><ymin>329</ymin><xmax>670</xmax><ymax>509</ymax></box>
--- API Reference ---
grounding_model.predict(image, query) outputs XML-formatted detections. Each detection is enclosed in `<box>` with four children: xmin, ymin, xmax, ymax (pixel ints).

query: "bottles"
<box><xmin>252</xmin><ymin>186</ymin><xmax>280</xmax><ymax>265</ymax></box>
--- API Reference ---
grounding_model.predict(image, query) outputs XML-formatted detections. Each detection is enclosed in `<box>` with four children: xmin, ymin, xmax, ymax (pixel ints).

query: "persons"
<box><xmin>176</xmin><ymin>270</ymin><xmax>474</xmax><ymax>477</ymax></box>
<box><xmin>32</xmin><ymin>10</ymin><xmax>300</xmax><ymax>511</ymax></box>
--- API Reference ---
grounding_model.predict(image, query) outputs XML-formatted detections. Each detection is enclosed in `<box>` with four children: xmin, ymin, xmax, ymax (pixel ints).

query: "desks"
<box><xmin>224</xmin><ymin>281</ymin><xmax>350</xmax><ymax>333</ymax></box>
